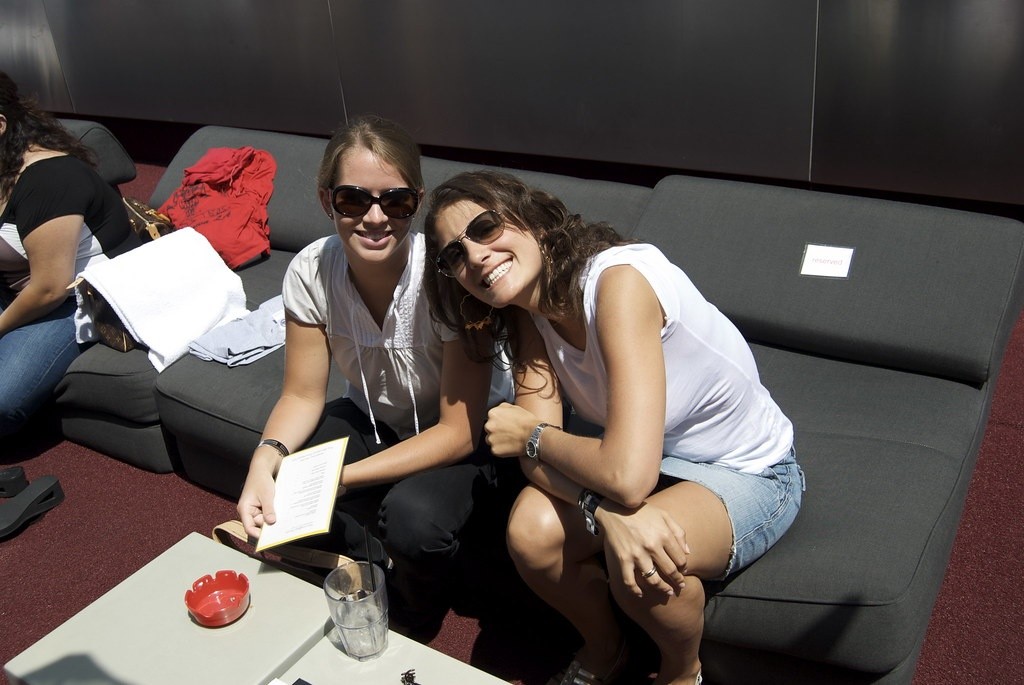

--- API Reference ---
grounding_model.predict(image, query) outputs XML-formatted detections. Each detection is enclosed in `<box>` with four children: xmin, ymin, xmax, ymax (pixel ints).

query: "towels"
<box><xmin>75</xmin><ymin>226</ymin><xmax>252</xmax><ymax>373</ymax></box>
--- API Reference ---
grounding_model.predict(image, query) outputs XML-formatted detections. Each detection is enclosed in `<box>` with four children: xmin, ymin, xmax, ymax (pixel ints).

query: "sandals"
<box><xmin>695</xmin><ymin>668</ymin><xmax>702</xmax><ymax>685</ymax></box>
<box><xmin>546</xmin><ymin>639</ymin><xmax>629</xmax><ymax>685</ymax></box>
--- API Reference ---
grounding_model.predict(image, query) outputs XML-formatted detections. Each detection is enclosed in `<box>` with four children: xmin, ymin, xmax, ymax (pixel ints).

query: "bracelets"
<box><xmin>577</xmin><ymin>488</ymin><xmax>604</xmax><ymax>536</ymax></box>
<box><xmin>257</xmin><ymin>439</ymin><xmax>289</xmax><ymax>456</ymax></box>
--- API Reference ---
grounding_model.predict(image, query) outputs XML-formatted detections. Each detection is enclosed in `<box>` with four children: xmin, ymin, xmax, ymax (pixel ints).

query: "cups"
<box><xmin>323</xmin><ymin>562</ymin><xmax>388</xmax><ymax>663</ymax></box>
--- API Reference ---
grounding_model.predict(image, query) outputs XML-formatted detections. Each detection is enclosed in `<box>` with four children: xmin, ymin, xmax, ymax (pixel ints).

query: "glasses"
<box><xmin>435</xmin><ymin>210</ymin><xmax>505</xmax><ymax>278</ymax></box>
<box><xmin>330</xmin><ymin>184</ymin><xmax>419</xmax><ymax>220</ymax></box>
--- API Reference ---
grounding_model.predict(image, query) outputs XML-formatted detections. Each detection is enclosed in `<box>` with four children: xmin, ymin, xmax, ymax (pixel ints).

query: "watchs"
<box><xmin>526</xmin><ymin>424</ymin><xmax>563</xmax><ymax>464</ymax></box>
<box><xmin>336</xmin><ymin>484</ymin><xmax>347</xmax><ymax>497</ymax></box>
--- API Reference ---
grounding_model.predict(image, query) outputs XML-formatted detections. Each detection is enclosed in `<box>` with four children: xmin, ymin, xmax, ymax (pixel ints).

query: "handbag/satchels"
<box><xmin>65</xmin><ymin>277</ymin><xmax>138</xmax><ymax>354</ymax></box>
<box><xmin>122</xmin><ymin>196</ymin><xmax>176</xmax><ymax>243</ymax></box>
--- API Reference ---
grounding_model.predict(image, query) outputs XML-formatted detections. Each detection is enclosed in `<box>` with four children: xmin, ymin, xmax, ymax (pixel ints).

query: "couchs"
<box><xmin>13</xmin><ymin>117</ymin><xmax>1024</xmax><ymax>685</ymax></box>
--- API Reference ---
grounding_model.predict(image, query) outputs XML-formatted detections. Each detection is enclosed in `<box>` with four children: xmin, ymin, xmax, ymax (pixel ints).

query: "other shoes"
<box><xmin>0</xmin><ymin>477</ymin><xmax>65</xmax><ymax>543</ymax></box>
<box><xmin>0</xmin><ymin>466</ymin><xmax>28</xmax><ymax>498</ymax></box>
<box><xmin>373</xmin><ymin>537</ymin><xmax>394</xmax><ymax>578</ymax></box>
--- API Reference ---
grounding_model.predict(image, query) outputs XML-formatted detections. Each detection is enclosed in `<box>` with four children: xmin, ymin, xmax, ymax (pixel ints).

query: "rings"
<box><xmin>642</xmin><ymin>566</ymin><xmax>657</xmax><ymax>578</ymax></box>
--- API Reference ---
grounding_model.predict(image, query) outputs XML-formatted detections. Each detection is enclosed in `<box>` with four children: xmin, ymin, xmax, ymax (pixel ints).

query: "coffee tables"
<box><xmin>2</xmin><ymin>531</ymin><xmax>513</xmax><ymax>685</ymax></box>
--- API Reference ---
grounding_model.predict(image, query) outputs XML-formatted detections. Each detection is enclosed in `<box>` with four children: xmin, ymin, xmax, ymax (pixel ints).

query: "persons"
<box><xmin>422</xmin><ymin>169</ymin><xmax>803</xmax><ymax>685</ymax></box>
<box><xmin>235</xmin><ymin>115</ymin><xmax>518</xmax><ymax>640</ymax></box>
<box><xmin>0</xmin><ymin>72</ymin><xmax>145</xmax><ymax>444</ymax></box>
